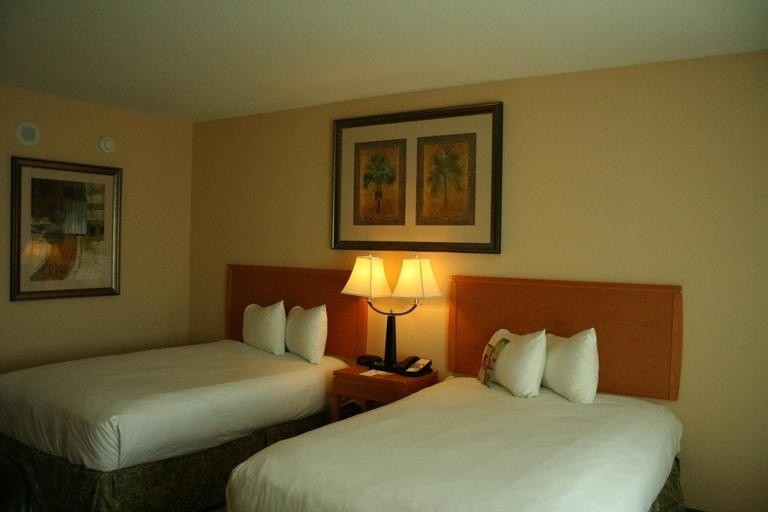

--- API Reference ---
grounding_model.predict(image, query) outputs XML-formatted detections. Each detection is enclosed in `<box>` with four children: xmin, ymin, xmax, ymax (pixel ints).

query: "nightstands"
<box><xmin>330</xmin><ymin>366</ymin><xmax>438</xmax><ymax>421</ymax></box>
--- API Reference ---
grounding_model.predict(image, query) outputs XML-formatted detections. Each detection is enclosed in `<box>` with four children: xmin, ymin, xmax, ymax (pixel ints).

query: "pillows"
<box><xmin>241</xmin><ymin>297</ymin><xmax>287</xmax><ymax>356</ymax></box>
<box><xmin>285</xmin><ymin>301</ymin><xmax>330</xmax><ymax>363</ymax></box>
<box><xmin>540</xmin><ymin>325</ymin><xmax>602</xmax><ymax>406</ymax></box>
<box><xmin>477</xmin><ymin>324</ymin><xmax>552</xmax><ymax>398</ymax></box>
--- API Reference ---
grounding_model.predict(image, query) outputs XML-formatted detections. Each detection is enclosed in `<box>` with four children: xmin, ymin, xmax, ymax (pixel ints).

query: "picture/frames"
<box><xmin>8</xmin><ymin>152</ymin><xmax>125</xmax><ymax>303</ymax></box>
<box><xmin>327</xmin><ymin>98</ymin><xmax>505</xmax><ymax>257</ymax></box>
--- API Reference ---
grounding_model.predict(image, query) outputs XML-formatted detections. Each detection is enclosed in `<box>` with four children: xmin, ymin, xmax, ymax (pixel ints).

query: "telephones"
<box><xmin>398</xmin><ymin>355</ymin><xmax>432</xmax><ymax>376</ymax></box>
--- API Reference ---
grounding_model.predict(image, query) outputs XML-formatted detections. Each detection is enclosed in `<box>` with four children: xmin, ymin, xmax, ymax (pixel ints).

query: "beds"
<box><xmin>1</xmin><ymin>261</ymin><xmax>373</xmax><ymax>512</ymax></box>
<box><xmin>221</xmin><ymin>271</ymin><xmax>694</xmax><ymax>512</ymax></box>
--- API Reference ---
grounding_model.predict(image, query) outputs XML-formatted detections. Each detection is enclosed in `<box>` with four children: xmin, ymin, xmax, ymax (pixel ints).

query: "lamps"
<box><xmin>339</xmin><ymin>251</ymin><xmax>444</xmax><ymax>373</ymax></box>
